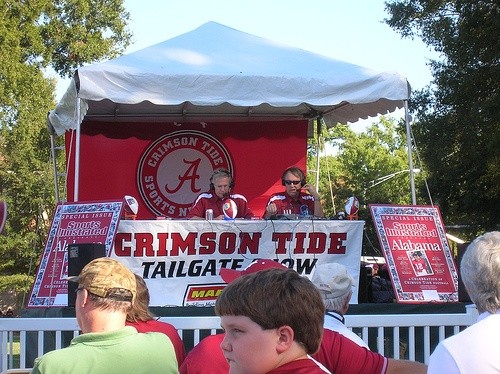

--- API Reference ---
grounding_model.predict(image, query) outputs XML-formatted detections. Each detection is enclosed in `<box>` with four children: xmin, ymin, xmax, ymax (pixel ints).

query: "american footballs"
<box><xmin>122</xmin><ymin>195</ymin><xmax>139</xmax><ymax>215</ymax></box>
<box><xmin>345</xmin><ymin>196</ymin><xmax>360</xmax><ymax>216</ymax></box>
<box><xmin>222</xmin><ymin>198</ymin><xmax>237</xmax><ymax>219</ymax></box>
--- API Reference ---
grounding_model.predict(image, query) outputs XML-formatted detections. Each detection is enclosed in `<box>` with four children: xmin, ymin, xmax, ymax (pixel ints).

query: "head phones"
<box><xmin>209</xmin><ymin>171</ymin><xmax>233</xmax><ymax>191</ymax></box>
<box><xmin>281</xmin><ymin>168</ymin><xmax>306</xmax><ymax>187</ymax></box>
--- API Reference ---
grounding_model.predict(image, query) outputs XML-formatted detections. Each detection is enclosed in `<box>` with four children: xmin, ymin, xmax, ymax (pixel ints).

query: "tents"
<box><xmin>46</xmin><ymin>21</ymin><xmax>417</xmax><ymax>205</ymax></box>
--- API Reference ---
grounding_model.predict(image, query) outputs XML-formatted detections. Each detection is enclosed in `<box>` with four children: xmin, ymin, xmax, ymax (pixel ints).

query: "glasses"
<box><xmin>285</xmin><ymin>181</ymin><xmax>301</xmax><ymax>185</ymax></box>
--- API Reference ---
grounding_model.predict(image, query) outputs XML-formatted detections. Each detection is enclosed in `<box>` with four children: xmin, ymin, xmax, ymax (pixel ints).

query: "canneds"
<box><xmin>205</xmin><ymin>209</ymin><xmax>213</xmax><ymax>221</ymax></box>
<box><xmin>337</xmin><ymin>211</ymin><xmax>345</xmax><ymax>219</ymax></box>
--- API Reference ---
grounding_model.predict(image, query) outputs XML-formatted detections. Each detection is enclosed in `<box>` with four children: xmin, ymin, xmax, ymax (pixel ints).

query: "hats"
<box><xmin>220</xmin><ymin>259</ymin><xmax>286</xmax><ymax>284</ymax></box>
<box><xmin>62</xmin><ymin>257</ymin><xmax>136</xmax><ymax>303</ymax></box>
<box><xmin>309</xmin><ymin>262</ymin><xmax>357</xmax><ymax>299</ymax></box>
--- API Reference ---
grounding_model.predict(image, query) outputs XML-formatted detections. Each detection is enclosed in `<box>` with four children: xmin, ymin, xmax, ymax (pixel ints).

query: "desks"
<box><xmin>110</xmin><ymin>219</ymin><xmax>365</xmax><ymax>308</ymax></box>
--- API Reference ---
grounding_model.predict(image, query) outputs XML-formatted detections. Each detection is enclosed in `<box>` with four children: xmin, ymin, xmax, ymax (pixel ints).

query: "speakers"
<box><xmin>67</xmin><ymin>243</ymin><xmax>105</xmax><ymax>307</ymax></box>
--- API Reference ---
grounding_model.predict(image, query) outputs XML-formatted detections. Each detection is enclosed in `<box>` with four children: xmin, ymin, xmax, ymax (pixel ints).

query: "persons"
<box><xmin>187</xmin><ymin>167</ymin><xmax>256</xmax><ymax>220</ymax></box>
<box><xmin>30</xmin><ymin>258</ymin><xmax>180</xmax><ymax>374</ymax></box>
<box><xmin>262</xmin><ymin>167</ymin><xmax>325</xmax><ymax>221</ymax></box>
<box><xmin>179</xmin><ymin>259</ymin><xmax>428</xmax><ymax>373</ymax></box>
<box><xmin>412</xmin><ymin>254</ymin><xmax>429</xmax><ymax>275</ymax></box>
<box><xmin>215</xmin><ymin>267</ymin><xmax>333</xmax><ymax>374</ymax></box>
<box><xmin>125</xmin><ymin>273</ymin><xmax>185</xmax><ymax>374</ymax></box>
<box><xmin>428</xmin><ymin>231</ymin><xmax>500</xmax><ymax>374</ymax></box>
<box><xmin>311</xmin><ymin>263</ymin><xmax>371</xmax><ymax>350</ymax></box>
